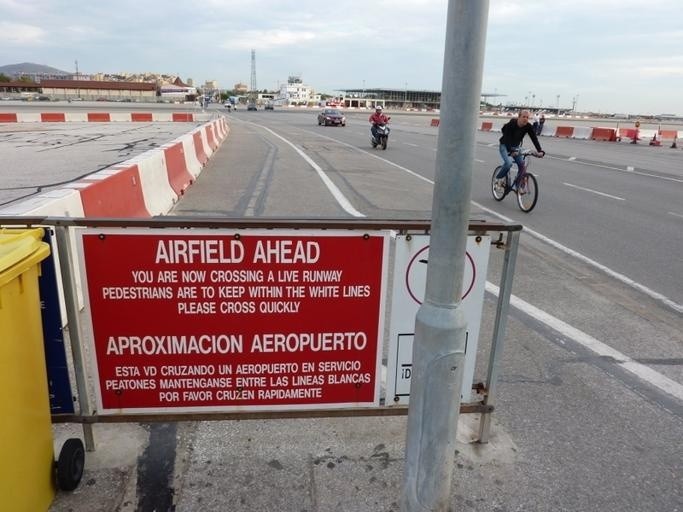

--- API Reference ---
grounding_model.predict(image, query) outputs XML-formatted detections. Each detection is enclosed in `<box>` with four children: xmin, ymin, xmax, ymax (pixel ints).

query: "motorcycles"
<box><xmin>370</xmin><ymin>117</ymin><xmax>391</xmax><ymax>150</ymax></box>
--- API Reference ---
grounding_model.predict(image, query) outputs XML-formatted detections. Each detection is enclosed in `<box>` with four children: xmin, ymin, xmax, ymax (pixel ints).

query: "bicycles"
<box><xmin>491</xmin><ymin>147</ymin><xmax>545</xmax><ymax>213</ymax></box>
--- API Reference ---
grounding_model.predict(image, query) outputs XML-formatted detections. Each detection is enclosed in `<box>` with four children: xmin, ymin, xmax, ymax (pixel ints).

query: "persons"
<box><xmin>538</xmin><ymin>115</ymin><xmax>545</xmax><ymax>136</ymax></box>
<box><xmin>496</xmin><ymin>110</ymin><xmax>545</xmax><ymax>196</ymax></box>
<box><xmin>369</xmin><ymin>106</ymin><xmax>390</xmax><ymax>139</ymax></box>
<box><xmin>533</xmin><ymin>113</ymin><xmax>540</xmax><ymax>135</ymax></box>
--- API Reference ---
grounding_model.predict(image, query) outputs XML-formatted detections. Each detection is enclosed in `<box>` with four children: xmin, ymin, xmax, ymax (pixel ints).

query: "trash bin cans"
<box><xmin>0</xmin><ymin>228</ymin><xmax>85</xmax><ymax>512</ymax></box>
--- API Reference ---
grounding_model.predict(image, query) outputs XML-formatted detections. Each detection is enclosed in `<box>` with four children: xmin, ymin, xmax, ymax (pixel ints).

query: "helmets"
<box><xmin>376</xmin><ymin>106</ymin><xmax>382</xmax><ymax>110</ymax></box>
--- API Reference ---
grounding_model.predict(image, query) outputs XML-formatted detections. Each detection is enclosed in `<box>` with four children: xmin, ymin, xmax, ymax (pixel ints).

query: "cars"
<box><xmin>264</xmin><ymin>102</ymin><xmax>273</xmax><ymax>110</ymax></box>
<box><xmin>224</xmin><ymin>100</ymin><xmax>231</xmax><ymax>108</ymax></box>
<box><xmin>317</xmin><ymin>108</ymin><xmax>346</xmax><ymax>127</ymax></box>
<box><xmin>247</xmin><ymin>104</ymin><xmax>257</xmax><ymax>112</ymax></box>
